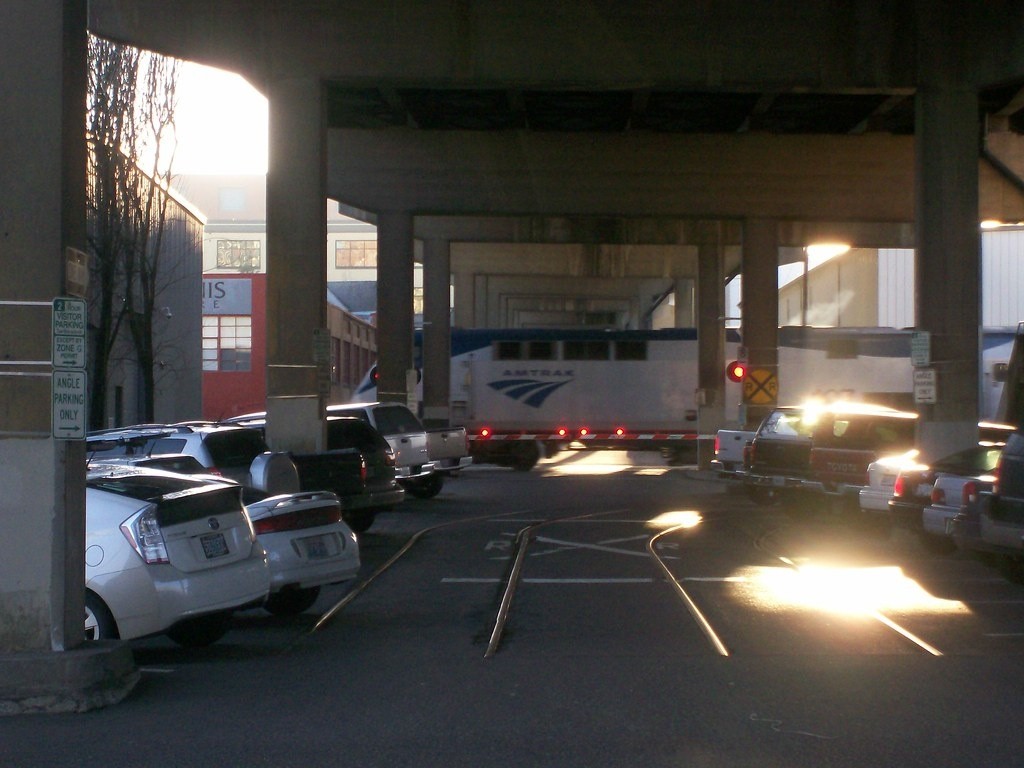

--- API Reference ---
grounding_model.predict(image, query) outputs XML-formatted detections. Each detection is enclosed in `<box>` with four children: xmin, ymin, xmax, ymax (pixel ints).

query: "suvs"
<box><xmin>84</xmin><ymin>421</ymin><xmax>300</xmax><ymax>495</ymax></box>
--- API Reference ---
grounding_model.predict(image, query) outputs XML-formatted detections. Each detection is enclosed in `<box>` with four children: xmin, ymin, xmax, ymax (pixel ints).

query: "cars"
<box><xmin>744</xmin><ymin>407</ymin><xmax>818</xmax><ymax>490</ymax></box>
<box><xmin>87</xmin><ymin>453</ymin><xmax>211</xmax><ymax>476</ymax></box>
<box><xmin>243</xmin><ymin>486</ymin><xmax>362</xmax><ymax>616</ymax></box>
<box><xmin>220</xmin><ymin>401</ymin><xmax>437</xmax><ymax>482</ymax></box>
<box><xmin>857</xmin><ymin>425</ymin><xmax>1024</xmax><ymax>549</ymax></box>
<box><xmin>803</xmin><ymin>408</ymin><xmax>916</xmax><ymax>505</ymax></box>
<box><xmin>236</xmin><ymin>417</ymin><xmax>407</xmax><ymax>531</ymax></box>
<box><xmin>710</xmin><ymin>429</ymin><xmax>758</xmax><ymax>479</ymax></box>
<box><xmin>85</xmin><ymin>464</ymin><xmax>271</xmax><ymax>647</ymax></box>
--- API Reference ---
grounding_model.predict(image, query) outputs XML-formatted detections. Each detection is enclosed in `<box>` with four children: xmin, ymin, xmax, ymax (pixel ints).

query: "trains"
<box><xmin>350</xmin><ymin>323</ymin><xmax>1024</xmax><ymax>469</ymax></box>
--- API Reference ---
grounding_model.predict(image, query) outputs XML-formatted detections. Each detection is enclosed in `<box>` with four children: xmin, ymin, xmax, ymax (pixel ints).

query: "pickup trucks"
<box><xmin>286</xmin><ymin>448</ymin><xmax>366</xmax><ymax>513</ymax></box>
<box><xmin>394</xmin><ymin>425</ymin><xmax>472</xmax><ymax>497</ymax></box>
